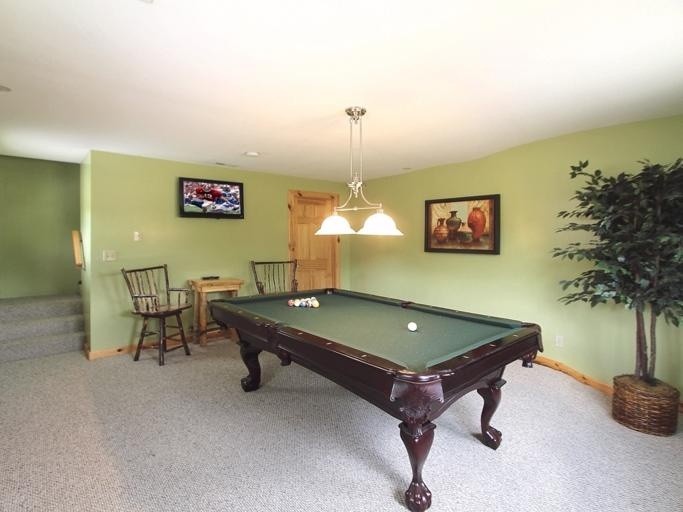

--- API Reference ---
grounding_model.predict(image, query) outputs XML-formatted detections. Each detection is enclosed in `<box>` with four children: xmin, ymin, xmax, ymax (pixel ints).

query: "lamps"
<box><xmin>312</xmin><ymin>105</ymin><xmax>404</xmax><ymax>239</ymax></box>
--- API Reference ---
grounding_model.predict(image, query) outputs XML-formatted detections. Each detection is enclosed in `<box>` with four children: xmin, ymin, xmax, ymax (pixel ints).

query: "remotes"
<box><xmin>202</xmin><ymin>276</ymin><xmax>220</xmax><ymax>279</ymax></box>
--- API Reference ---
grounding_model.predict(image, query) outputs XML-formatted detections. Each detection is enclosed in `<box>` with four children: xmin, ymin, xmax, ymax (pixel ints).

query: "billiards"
<box><xmin>288</xmin><ymin>297</ymin><xmax>320</xmax><ymax>308</ymax></box>
<box><xmin>408</xmin><ymin>322</ymin><xmax>417</xmax><ymax>331</ymax></box>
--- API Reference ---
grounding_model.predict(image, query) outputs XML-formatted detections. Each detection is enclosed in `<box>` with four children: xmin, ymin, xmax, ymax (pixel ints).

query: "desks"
<box><xmin>204</xmin><ymin>287</ymin><xmax>544</xmax><ymax>511</ymax></box>
<box><xmin>189</xmin><ymin>278</ymin><xmax>244</xmax><ymax>347</ymax></box>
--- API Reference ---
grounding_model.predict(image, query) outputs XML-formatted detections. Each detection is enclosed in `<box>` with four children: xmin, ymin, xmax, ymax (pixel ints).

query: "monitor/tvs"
<box><xmin>178</xmin><ymin>176</ymin><xmax>244</xmax><ymax>219</ymax></box>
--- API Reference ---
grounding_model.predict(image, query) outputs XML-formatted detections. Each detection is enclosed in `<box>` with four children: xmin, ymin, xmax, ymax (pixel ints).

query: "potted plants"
<box><xmin>548</xmin><ymin>155</ymin><xmax>683</xmax><ymax>436</ymax></box>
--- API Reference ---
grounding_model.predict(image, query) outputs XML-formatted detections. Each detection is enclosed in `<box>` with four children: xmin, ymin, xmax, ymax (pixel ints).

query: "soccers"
<box><xmin>211</xmin><ymin>205</ymin><xmax>216</xmax><ymax>209</ymax></box>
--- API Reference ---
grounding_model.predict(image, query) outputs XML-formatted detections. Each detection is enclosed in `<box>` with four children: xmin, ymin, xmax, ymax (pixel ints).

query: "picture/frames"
<box><xmin>423</xmin><ymin>193</ymin><xmax>502</xmax><ymax>256</ymax></box>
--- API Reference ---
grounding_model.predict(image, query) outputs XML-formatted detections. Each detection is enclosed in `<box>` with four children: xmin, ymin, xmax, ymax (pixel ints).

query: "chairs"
<box><xmin>249</xmin><ymin>259</ymin><xmax>298</xmax><ymax>296</ymax></box>
<box><xmin>119</xmin><ymin>263</ymin><xmax>191</xmax><ymax>366</ymax></box>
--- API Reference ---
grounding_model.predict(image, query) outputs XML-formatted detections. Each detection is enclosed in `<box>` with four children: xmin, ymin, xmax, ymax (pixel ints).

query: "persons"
<box><xmin>183</xmin><ymin>182</ymin><xmax>240</xmax><ymax>213</ymax></box>
